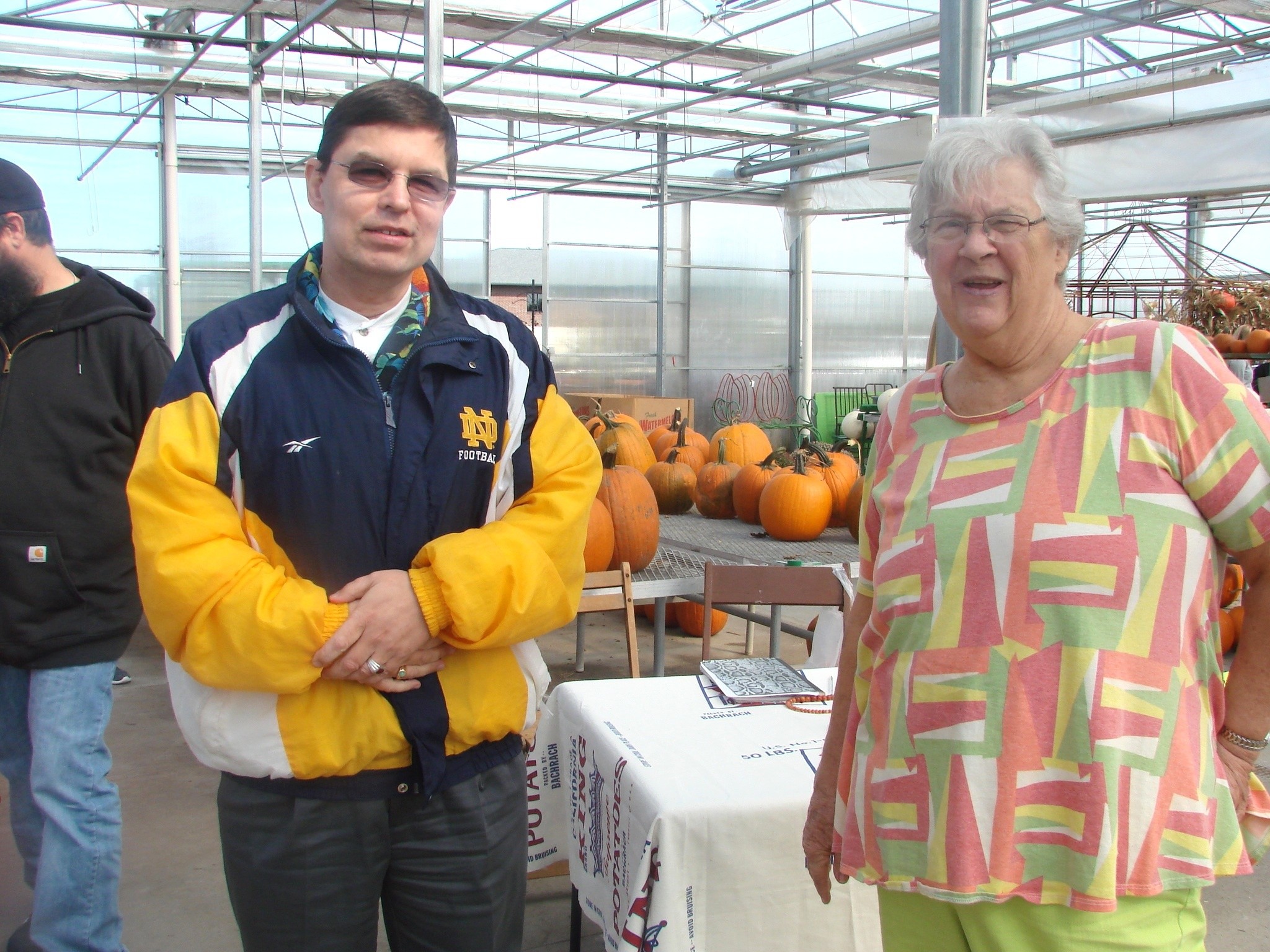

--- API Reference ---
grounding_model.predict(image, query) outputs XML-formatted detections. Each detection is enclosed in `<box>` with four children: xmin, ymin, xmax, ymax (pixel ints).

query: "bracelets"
<box><xmin>1219</xmin><ymin>726</ymin><xmax>1270</xmax><ymax>751</ymax></box>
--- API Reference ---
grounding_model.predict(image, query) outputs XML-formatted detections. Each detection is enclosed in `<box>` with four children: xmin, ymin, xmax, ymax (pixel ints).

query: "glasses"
<box><xmin>920</xmin><ymin>213</ymin><xmax>1044</xmax><ymax>243</ymax></box>
<box><xmin>325</xmin><ymin>159</ymin><xmax>455</xmax><ymax>202</ymax></box>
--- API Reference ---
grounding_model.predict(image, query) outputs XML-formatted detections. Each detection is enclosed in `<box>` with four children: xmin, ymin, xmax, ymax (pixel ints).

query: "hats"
<box><xmin>0</xmin><ymin>158</ymin><xmax>46</xmax><ymax>214</ymax></box>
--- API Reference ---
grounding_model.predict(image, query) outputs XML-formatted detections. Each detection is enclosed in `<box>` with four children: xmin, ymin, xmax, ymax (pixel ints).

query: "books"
<box><xmin>699</xmin><ymin>657</ymin><xmax>825</xmax><ymax>704</ymax></box>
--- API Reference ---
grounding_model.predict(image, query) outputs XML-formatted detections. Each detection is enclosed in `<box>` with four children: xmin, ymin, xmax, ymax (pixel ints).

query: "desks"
<box><xmin>577</xmin><ymin>511</ymin><xmax>862</xmax><ymax>679</ymax></box>
<box><xmin>527</xmin><ymin>666</ymin><xmax>884</xmax><ymax>951</ymax></box>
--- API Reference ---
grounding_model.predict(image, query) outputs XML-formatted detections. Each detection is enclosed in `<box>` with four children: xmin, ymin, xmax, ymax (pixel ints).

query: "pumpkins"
<box><xmin>633</xmin><ymin>601</ymin><xmax>728</xmax><ymax>638</ymax></box>
<box><xmin>1204</xmin><ymin>290</ymin><xmax>1270</xmax><ymax>353</ymax></box>
<box><xmin>577</xmin><ymin>398</ymin><xmax>865</xmax><ymax>572</ymax></box>
<box><xmin>1218</xmin><ymin>564</ymin><xmax>1245</xmax><ymax>653</ymax></box>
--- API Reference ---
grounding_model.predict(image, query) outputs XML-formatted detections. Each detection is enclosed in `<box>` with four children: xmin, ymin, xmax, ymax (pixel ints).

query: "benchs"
<box><xmin>712</xmin><ymin>372</ymin><xmax>822</xmax><ymax>449</ymax></box>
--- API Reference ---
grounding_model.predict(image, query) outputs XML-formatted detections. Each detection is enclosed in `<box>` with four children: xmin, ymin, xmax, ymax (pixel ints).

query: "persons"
<box><xmin>1225</xmin><ymin>358</ymin><xmax>1270</xmax><ymax>408</ymax></box>
<box><xmin>0</xmin><ymin>157</ymin><xmax>176</xmax><ymax>952</ymax></box>
<box><xmin>125</xmin><ymin>85</ymin><xmax>605</xmax><ymax>950</ymax></box>
<box><xmin>805</xmin><ymin>112</ymin><xmax>1269</xmax><ymax>949</ymax></box>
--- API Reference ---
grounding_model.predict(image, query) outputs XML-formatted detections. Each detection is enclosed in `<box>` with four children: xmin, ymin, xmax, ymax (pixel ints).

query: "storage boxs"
<box><xmin>565</xmin><ymin>393</ymin><xmax>694</xmax><ymax>437</ymax></box>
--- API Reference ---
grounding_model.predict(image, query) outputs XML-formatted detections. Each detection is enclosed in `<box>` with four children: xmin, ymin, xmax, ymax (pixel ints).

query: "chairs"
<box><xmin>528</xmin><ymin>562</ymin><xmax>641</xmax><ymax>881</ymax></box>
<box><xmin>832</xmin><ymin>383</ymin><xmax>893</xmax><ymax>475</ymax></box>
<box><xmin>702</xmin><ymin>561</ymin><xmax>852</xmax><ymax>674</ymax></box>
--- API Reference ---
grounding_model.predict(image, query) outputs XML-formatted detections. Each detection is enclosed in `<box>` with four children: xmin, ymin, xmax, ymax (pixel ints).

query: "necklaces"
<box><xmin>785</xmin><ymin>694</ymin><xmax>833</xmax><ymax>714</ymax></box>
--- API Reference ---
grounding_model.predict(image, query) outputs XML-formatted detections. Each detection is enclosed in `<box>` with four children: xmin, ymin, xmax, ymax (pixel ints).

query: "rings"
<box><xmin>396</xmin><ymin>664</ymin><xmax>406</xmax><ymax>680</ymax></box>
<box><xmin>368</xmin><ymin>659</ymin><xmax>385</xmax><ymax>676</ymax></box>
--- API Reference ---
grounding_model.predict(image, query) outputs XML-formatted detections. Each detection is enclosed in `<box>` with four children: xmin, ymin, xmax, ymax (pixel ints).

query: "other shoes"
<box><xmin>112</xmin><ymin>666</ymin><xmax>131</xmax><ymax>685</ymax></box>
